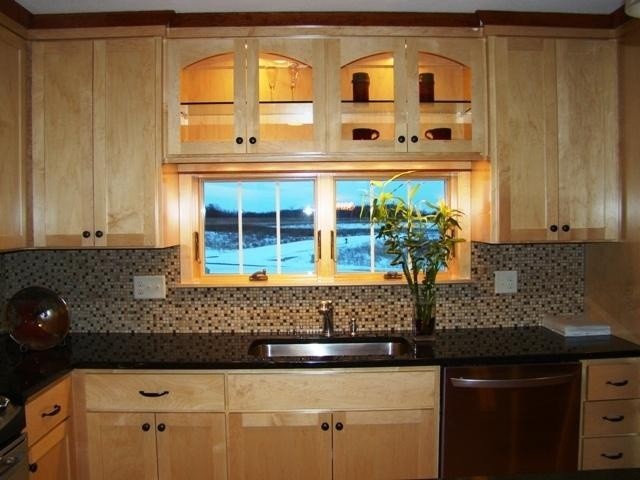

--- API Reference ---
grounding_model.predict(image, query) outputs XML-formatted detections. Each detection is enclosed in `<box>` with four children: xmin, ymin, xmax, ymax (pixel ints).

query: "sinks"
<box><xmin>248</xmin><ymin>336</ymin><xmax>411</xmax><ymax>357</ymax></box>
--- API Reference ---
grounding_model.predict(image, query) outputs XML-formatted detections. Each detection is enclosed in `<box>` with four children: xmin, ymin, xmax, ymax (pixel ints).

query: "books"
<box><xmin>541</xmin><ymin>315</ymin><xmax>612</xmax><ymax>339</ymax></box>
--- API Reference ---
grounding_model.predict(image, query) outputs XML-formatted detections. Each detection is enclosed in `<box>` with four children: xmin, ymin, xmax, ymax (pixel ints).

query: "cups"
<box><xmin>425</xmin><ymin>127</ymin><xmax>451</xmax><ymax>140</ymax></box>
<box><xmin>352</xmin><ymin>128</ymin><xmax>380</xmax><ymax>139</ymax></box>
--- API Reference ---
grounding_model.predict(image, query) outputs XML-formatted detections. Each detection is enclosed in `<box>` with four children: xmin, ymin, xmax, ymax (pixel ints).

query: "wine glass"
<box><xmin>263</xmin><ymin>65</ymin><xmax>278</xmax><ymax>101</ymax></box>
<box><xmin>288</xmin><ymin>62</ymin><xmax>299</xmax><ymax>102</ymax></box>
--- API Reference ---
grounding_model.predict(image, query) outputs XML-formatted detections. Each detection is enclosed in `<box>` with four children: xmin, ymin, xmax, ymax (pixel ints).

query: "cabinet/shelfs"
<box><xmin>224</xmin><ymin>363</ymin><xmax>441</xmax><ymax>480</ymax></box>
<box><xmin>452</xmin><ymin>18</ymin><xmax>625</xmax><ymax>244</ymax></box>
<box><xmin>72</xmin><ymin>364</ymin><xmax>226</xmax><ymax>480</ymax></box>
<box><xmin>29</xmin><ymin>21</ymin><xmax>181</xmax><ymax>250</ymax></box>
<box><xmin>575</xmin><ymin>352</ymin><xmax>640</xmax><ymax>474</ymax></box>
<box><xmin>0</xmin><ymin>12</ymin><xmax>29</xmax><ymax>253</ymax></box>
<box><xmin>22</xmin><ymin>367</ymin><xmax>81</xmax><ymax>480</ymax></box>
<box><xmin>579</xmin><ymin>15</ymin><xmax>638</xmax><ymax>346</ymax></box>
<box><xmin>161</xmin><ymin>20</ymin><xmax>332</xmax><ymax>166</ymax></box>
<box><xmin>332</xmin><ymin>22</ymin><xmax>492</xmax><ymax>167</ymax></box>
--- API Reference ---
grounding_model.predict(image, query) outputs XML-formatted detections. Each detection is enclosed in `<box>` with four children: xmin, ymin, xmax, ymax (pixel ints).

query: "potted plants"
<box><xmin>354</xmin><ymin>165</ymin><xmax>471</xmax><ymax>343</ymax></box>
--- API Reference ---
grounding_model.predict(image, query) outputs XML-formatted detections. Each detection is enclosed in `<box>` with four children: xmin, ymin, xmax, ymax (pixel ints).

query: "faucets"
<box><xmin>318</xmin><ymin>299</ymin><xmax>335</xmax><ymax>337</ymax></box>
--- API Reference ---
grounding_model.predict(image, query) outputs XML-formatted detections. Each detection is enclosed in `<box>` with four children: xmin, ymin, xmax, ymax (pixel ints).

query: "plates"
<box><xmin>3</xmin><ymin>286</ymin><xmax>70</xmax><ymax>353</ymax></box>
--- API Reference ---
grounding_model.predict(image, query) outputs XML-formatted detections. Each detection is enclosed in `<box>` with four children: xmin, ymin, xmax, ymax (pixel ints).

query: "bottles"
<box><xmin>418</xmin><ymin>72</ymin><xmax>434</xmax><ymax>102</ymax></box>
<box><xmin>351</xmin><ymin>72</ymin><xmax>370</xmax><ymax>102</ymax></box>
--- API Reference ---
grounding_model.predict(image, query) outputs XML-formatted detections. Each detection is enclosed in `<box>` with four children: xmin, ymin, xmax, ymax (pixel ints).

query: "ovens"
<box><xmin>0</xmin><ymin>398</ymin><xmax>30</xmax><ymax>480</ymax></box>
